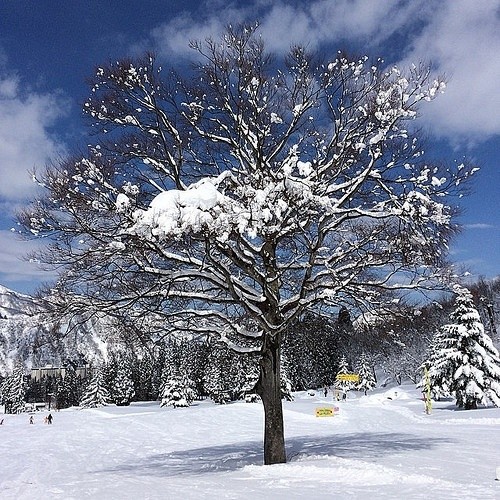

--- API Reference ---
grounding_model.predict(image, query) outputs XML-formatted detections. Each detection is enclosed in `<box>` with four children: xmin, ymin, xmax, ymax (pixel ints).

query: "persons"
<box><xmin>336</xmin><ymin>393</ymin><xmax>341</xmax><ymax>401</ymax></box>
<box><xmin>396</xmin><ymin>372</ymin><xmax>402</xmax><ymax>386</ymax></box>
<box><xmin>324</xmin><ymin>387</ymin><xmax>328</xmax><ymax>398</ymax></box>
<box><xmin>353</xmin><ymin>381</ymin><xmax>358</xmax><ymax>392</ymax></box>
<box><xmin>363</xmin><ymin>381</ymin><xmax>368</xmax><ymax>396</ymax></box>
<box><xmin>286</xmin><ymin>392</ymin><xmax>294</xmax><ymax>402</ymax></box>
<box><xmin>342</xmin><ymin>392</ymin><xmax>347</xmax><ymax>402</ymax></box>
<box><xmin>371</xmin><ymin>382</ymin><xmax>376</xmax><ymax>392</ymax></box>
